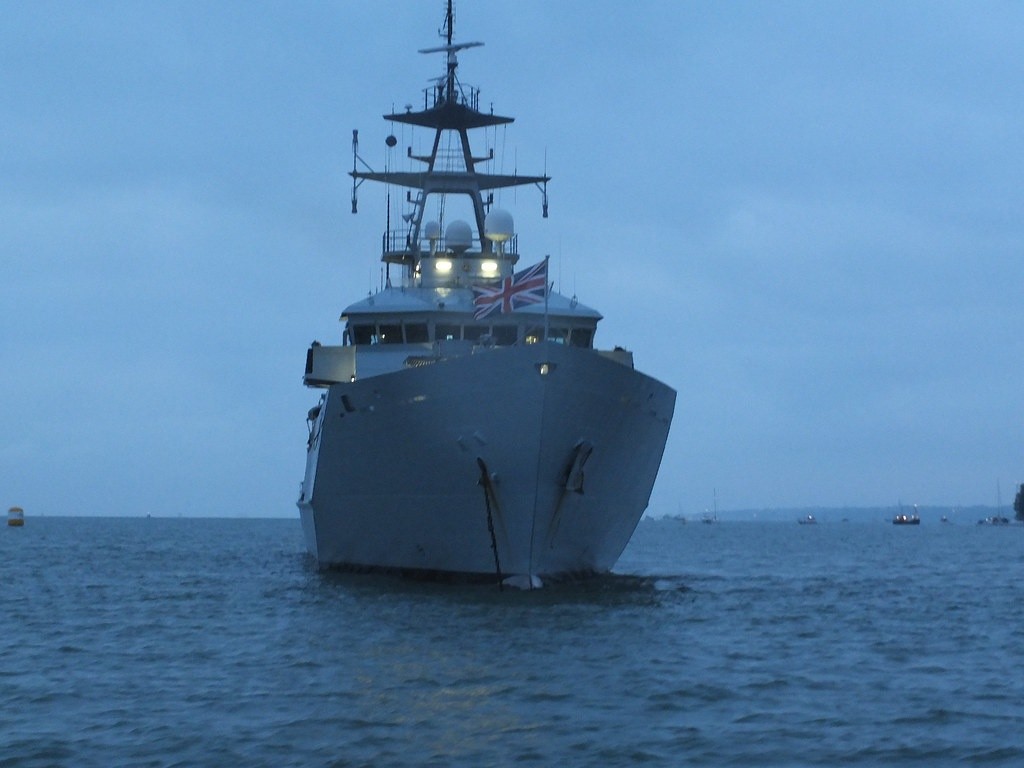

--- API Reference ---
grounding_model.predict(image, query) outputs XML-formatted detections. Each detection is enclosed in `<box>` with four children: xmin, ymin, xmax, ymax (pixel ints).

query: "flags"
<box><xmin>473</xmin><ymin>259</ymin><xmax>547</xmax><ymax>320</ymax></box>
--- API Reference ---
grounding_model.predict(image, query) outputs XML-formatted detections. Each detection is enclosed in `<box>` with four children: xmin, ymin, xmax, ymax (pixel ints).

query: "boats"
<box><xmin>892</xmin><ymin>504</ymin><xmax>920</xmax><ymax>524</ymax></box>
<box><xmin>8</xmin><ymin>507</ymin><xmax>24</xmax><ymax>526</ymax></box>
<box><xmin>798</xmin><ymin>513</ymin><xmax>817</xmax><ymax>524</ymax></box>
<box><xmin>646</xmin><ymin>502</ymin><xmax>685</xmax><ymax>521</ymax></box>
<box><xmin>295</xmin><ymin>0</ymin><xmax>676</xmax><ymax>575</ymax></box>
<box><xmin>977</xmin><ymin>478</ymin><xmax>1009</xmax><ymax>525</ymax></box>
<box><xmin>701</xmin><ymin>488</ymin><xmax>718</xmax><ymax>524</ymax></box>
